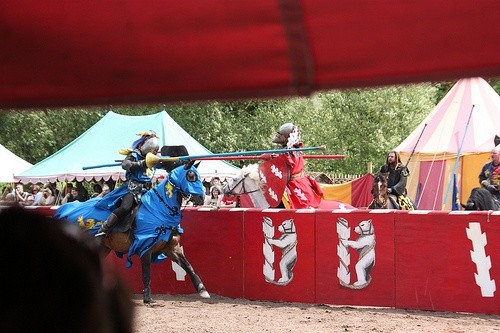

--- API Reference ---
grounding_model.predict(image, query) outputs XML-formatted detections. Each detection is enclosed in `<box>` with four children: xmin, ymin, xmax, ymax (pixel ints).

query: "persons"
<box><xmin>94</xmin><ymin>130</ymin><xmax>190</xmax><ymax>239</ymax></box>
<box><xmin>479</xmin><ymin>144</ymin><xmax>500</xmax><ymax>203</ymax></box>
<box><xmin>0</xmin><ymin>207</ymin><xmax>137</xmax><ymax>333</ymax></box>
<box><xmin>367</xmin><ymin>151</ymin><xmax>415</xmax><ymax>211</ymax></box>
<box><xmin>257</xmin><ymin>122</ymin><xmax>325</xmax><ymax>209</ymax></box>
<box><xmin>0</xmin><ymin>177</ymin><xmax>237</xmax><ymax>209</ymax></box>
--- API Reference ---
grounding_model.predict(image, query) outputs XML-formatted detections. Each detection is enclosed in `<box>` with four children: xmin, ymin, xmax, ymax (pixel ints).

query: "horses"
<box><xmin>53</xmin><ymin>159</ymin><xmax>211</xmax><ymax>303</ymax></box>
<box><xmin>368</xmin><ymin>171</ymin><xmax>418</xmax><ymax>209</ymax></box>
<box><xmin>220</xmin><ymin>164</ymin><xmax>360</xmax><ymax>209</ymax></box>
<box><xmin>461</xmin><ymin>187</ymin><xmax>500</xmax><ymax>211</ymax></box>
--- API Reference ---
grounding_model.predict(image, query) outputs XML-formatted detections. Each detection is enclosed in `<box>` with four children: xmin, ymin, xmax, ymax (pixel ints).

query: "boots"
<box><xmin>95</xmin><ymin>212</ymin><xmax>119</xmax><ymax>248</ymax></box>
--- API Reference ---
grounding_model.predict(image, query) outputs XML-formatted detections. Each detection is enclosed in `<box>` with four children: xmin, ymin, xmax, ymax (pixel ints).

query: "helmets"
<box><xmin>273</xmin><ymin>122</ymin><xmax>296</xmax><ymax>143</ymax></box>
<box><xmin>133</xmin><ymin>132</ymin><xmax>160</xmax><ymax>155</ymax></box>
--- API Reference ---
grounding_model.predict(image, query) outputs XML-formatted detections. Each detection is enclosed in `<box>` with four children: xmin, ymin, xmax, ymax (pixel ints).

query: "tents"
<box><xmin>389</xmin><ymin>75</ymin><xmax>500</xmax><ymax>211</ymax></box>
<box><xmin>14</xmin><ymin>110</ymin><xmax>244</xmax><ymax>186</ymax></box>
